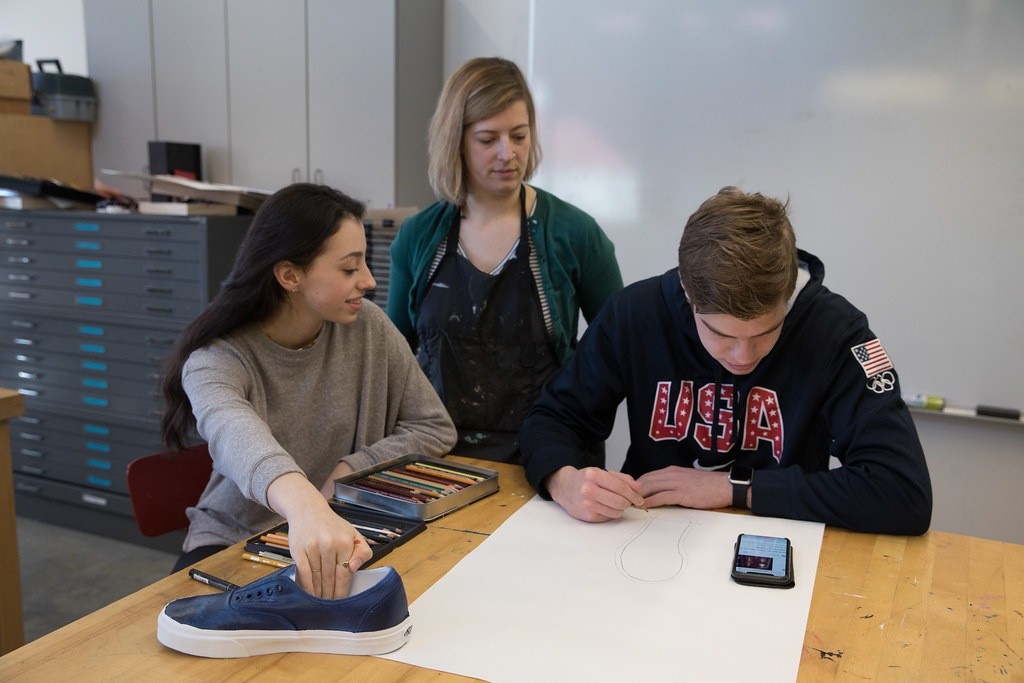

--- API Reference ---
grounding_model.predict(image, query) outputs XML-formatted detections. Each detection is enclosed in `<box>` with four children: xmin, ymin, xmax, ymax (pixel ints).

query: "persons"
<box><xmin>158</xmin><ymin>183</ymin><xmax>458</xmax><ymax>601</ymax></box>
<box><xmin>518</xmin><ymin>186</ymin><xmax>933</xmax><ymax>537</ymax></box>
<box><xmin>387</xmin><ymin>57</ymin><xmax>624</xmax><ymax>469</ymax></box>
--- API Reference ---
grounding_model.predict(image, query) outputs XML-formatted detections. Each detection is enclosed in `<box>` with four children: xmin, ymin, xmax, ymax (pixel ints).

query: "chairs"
<box><xmin>126</xmin><ymin>443</ymin><xmax>214</xmax><ymax>539</ymax></box>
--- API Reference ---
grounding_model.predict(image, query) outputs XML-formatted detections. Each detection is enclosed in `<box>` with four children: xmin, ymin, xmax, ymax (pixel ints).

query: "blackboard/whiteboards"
<box><xmin>529</xmin><ymin>0</ymin><xmax>1024</xmax><ymax>426</ymax></box>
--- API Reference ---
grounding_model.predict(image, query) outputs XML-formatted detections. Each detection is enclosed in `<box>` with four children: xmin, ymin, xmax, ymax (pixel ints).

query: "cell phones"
<box><xmin>730</xmin><ymin>534</ymin><xmax>791</xmax><ymax>585</ymax></box>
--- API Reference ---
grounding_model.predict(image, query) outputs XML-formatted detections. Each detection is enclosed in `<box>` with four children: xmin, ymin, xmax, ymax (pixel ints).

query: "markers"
<box><xmin>189</xmin><ymin>567</ymin><xmax>241</xmax><ymax>592</ymax></box>
<box><xmin>900</xmin><ymin>391</ymin><xmax>946</xmax><ymax>412</ymax></box>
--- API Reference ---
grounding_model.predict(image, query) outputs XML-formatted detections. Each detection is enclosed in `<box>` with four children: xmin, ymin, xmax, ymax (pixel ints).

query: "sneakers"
<box><xmin>155</xmin><ymin>564</ymin><xmax>412</xmax><ymax>659</ymax></box>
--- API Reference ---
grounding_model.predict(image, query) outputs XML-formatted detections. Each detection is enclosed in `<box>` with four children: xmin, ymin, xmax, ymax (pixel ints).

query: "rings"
<box><xmin>312</xmin><ymin>569</ymin><xmax>320</xmax><ymax>572</ymax></box>
<box><xmin>336</xmin><ymin>561</ymin><xmax>349</xmax><ymax>567</ymax></box>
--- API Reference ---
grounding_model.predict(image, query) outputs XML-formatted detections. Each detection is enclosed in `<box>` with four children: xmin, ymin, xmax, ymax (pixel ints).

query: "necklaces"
<box><xmin>259</xmin><ymin>325</ymin><xmax>319</xmax><ymax>350</ymax></box>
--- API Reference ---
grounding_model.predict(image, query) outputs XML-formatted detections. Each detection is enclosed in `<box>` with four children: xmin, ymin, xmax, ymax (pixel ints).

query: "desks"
<box><xmin>47</xmin><ymin>455</ymin><xmax>1023</xmax><ymax>683</ymax></box>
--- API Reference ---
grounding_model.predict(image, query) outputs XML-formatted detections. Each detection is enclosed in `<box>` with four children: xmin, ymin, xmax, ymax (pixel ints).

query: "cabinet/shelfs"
<box><xmin>1</xmin><ymin>187</ymin><xmax>254</xmax><ymax>557</ymax></box>
<box><xmin>84</xmin><ymin>0</ymin><xmax>444</xmax><ymax>221</ymax></box>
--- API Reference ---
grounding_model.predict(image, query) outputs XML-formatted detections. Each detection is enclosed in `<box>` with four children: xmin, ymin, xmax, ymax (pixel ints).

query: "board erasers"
<box><xmin>975</xmin><ymin>403</ymin><xmax>1020</xmax><ymax>420</ymax></box>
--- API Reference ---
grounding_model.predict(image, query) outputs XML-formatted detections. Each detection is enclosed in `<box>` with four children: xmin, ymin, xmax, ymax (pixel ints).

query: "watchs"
<box><xmin>728</xmin><ymin>466</ymin><xmax>755</xmax><ymax>507</ymax></box>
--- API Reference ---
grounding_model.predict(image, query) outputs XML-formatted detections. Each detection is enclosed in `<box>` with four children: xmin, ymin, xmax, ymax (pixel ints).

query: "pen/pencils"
<box><xmin>243</xmin><ymin>514</ymin><xmax>404</xmax><ymax>571</ymax></box>
<box><xmin>351</xmin><ymin>462</ymin><xmax>492</xmax><ymax>504</ymax></box>
<box><xmin>632</xmin><ymin>502</ymin><xmax>650</xmax><ymax>513</ymax></box>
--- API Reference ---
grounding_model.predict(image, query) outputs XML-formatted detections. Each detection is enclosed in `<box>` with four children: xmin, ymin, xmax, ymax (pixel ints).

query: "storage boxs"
<box><xmin>0</xmin><ymin>59</ymin><xmax>94</xmax><ymax>210</ymax></box>
<box><xmin>333</xmin><ymin>453</ymin><xmax>500</xmax><ymax>524</ymax></box>
